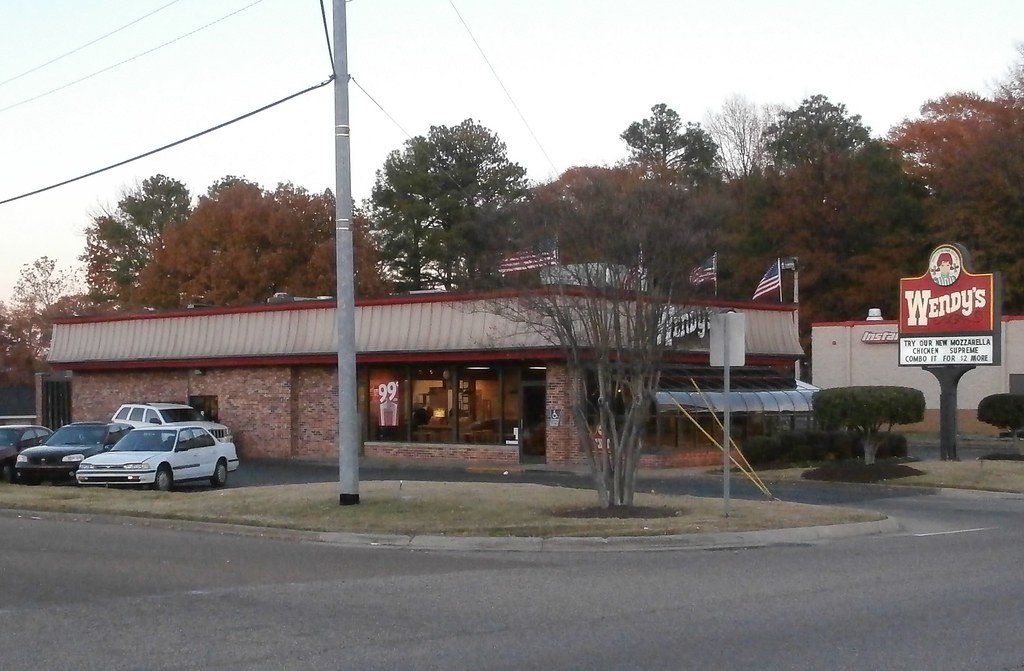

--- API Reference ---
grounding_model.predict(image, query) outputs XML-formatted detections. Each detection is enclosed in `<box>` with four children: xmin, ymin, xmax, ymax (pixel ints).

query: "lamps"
<box><xmin>194</xmin><ymin>369</ymin><xmax>204</xmax><ymax>375</ymax></box>
<box><xmin>65</xmin><ymin>370</ymin><xmax>79</xmax><ymax>377</ymax></box>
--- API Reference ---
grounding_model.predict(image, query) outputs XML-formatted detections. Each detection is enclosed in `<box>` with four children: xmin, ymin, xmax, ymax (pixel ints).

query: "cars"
<box><xmin>15</xmin><ymin>421</ymin><xmax>135</xmax><ymax>486</ymax></box>
<box><xmin>0</xmin><ymin>425</ymin><xmax>55</xmax><ymax>483</ymax></box>
<box><xmin>76</xmin><ymin>425</ymin><xmax>240</xmax><ymax>491</ymax></box>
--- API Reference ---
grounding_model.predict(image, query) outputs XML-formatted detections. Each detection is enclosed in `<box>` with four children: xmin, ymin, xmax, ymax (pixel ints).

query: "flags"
<box><xmin>497</xmin><ymin>236</ymin><xmax>558</xmax><ymax>272</ymax></box>
<box><xmin>752</xmin><ymin>260</ymin><xmax>779</xmax><ymax>300</ymax></box>
<box><xmin>626</xmin><ymin>253</ymin><xmax>641</xmax><ymax>284</ymax></box>
<box><xmin>690</xmin><ymin>254</ymin><xmax>715</xmax><ymax>286</ymax></box>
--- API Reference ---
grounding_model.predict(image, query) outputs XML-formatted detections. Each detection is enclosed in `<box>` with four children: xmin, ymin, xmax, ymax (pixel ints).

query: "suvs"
<box><xmin>106</xmin><ymin>402</ymin><xmax>233</xmax><ymax>444</ymax></box>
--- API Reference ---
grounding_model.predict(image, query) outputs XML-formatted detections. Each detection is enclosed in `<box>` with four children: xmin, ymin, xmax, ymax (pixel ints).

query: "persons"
<box><xmin>414</xmin><ymin>403</ymin><xmax>432</xmax><ymax>432</ymax></box>
